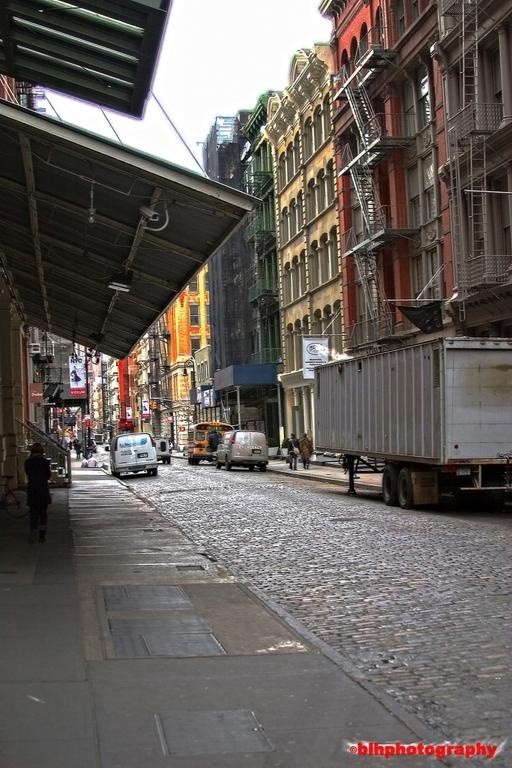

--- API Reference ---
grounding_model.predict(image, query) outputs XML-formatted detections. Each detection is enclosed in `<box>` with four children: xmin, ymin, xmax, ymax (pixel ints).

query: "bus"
<box><xmin>187</xmin><ymin>421</ymin><xmax>235</xmax><ymax>465</ymax></box>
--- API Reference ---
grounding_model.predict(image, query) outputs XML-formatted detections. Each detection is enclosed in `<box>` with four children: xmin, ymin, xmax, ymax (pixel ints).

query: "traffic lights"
<box><xmin>180</xmin><ymin>427</ymin><xmax>185</xmax><ymax>431</ymax></box>
<box><xmin>149</xmin><ymin>401</ymin><xmax>156</xmax><ymax>410</ymax></box>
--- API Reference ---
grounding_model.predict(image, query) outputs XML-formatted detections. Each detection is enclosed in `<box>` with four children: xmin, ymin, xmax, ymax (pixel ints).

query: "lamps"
<box><xmin>87</xmin><ymin>331</ymin><xmax>105</xmax><ymax>345</ymax></box>
<box><xmin>88</xmin><ymin>183</ymin><xmax>97</xmax><ymax>224</ymax></box>
<box><xmin>106</xmin><ymin>270</ymin><xmax>133</xmax><ymax>293</ymax></box>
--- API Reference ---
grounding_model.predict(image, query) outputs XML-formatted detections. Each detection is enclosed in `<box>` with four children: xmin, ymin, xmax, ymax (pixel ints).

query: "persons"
<box><xmin>24</xmin><ymin>442</ymin><xmax>53</xmax><ymax>545</ymax></box>
<box><xmin>208</xmin><ymin>429</ymin><xmax>220</xmax><ymax>451</ymax></box>
<box><xmin>64</xmin><ymin>438</ymin><xmax>98</xmax><ymax>459</ymax></box>
<box><xmin>299</xmin><ymin>433</ymin><xmax>313</xmax><ymax>469</ymax></box>
<box><xmin>70</xmin><ymin>365</ymin><xmax>81</xmax><ymax>388</ymax></box>
<box><xmin>281</xmin><ymin>438</ymin><xmax>288</xmax><ymax>448</ymax></box>
<box><xmin>286</xmin><ymin>433</ymin><xmax>300</xmax><ymax>471</ymax></box>
<box><xmin>285</xmin><ymin>437</ymin><xmax>291</xmax><ymax>448</ymax></box>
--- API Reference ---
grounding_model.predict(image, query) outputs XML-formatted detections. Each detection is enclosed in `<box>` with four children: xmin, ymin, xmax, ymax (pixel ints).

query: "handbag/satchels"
<box><xmin>311</xmin><ymin>454</ymin><xmax>316</xmax><ymax>461</ymax></box>
<box><xmin>285</xmin><ymin>456</ymin><xmax>291</xmax><ymax>464</ymax></box>
<box><xmin>294</xmin><ymin>448</ymin><xmax>299</xmax><ymax>454</ymax></box>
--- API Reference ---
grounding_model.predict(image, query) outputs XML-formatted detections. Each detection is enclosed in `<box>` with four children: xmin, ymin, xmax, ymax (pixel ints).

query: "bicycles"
<box><xmin>0</xmin><ymin>474</ymin><xmax>30</xmax><ymax>519</ymax></box>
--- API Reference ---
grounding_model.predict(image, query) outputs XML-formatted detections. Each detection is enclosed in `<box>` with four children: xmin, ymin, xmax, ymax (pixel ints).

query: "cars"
<box><xmin>110</xmin><ymin>432</ymin><xmax>171</xmax><ymax>476</ymax></box>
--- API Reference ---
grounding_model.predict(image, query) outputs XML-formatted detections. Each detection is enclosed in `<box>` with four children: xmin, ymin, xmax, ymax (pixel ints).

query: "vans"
<box><xmin>216</xmin><ymin>430</ymin><xmax>269</xmax><ymax>472</ymax></box>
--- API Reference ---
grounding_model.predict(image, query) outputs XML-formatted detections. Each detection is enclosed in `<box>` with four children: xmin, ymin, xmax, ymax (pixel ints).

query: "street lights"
<box><xmin>183</xmin><ymin>359</ymin><xmax>198</xmax><ymax>424</ymax></box>
